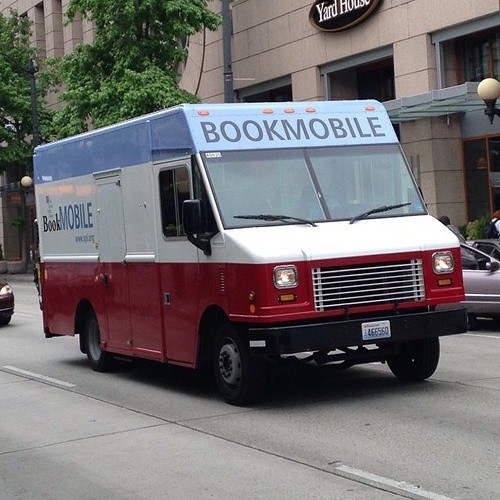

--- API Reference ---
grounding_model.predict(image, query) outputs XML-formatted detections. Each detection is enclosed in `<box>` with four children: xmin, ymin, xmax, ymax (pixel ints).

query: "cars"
<box><xmin>0</xmin><ymin>280</ymin><xmax>15</xmax><ymax>326</ymax></box>
<box><xmin>460</xmin><ymin>240</ymin><xmax>500</xmax><ymax>325</ymax></box>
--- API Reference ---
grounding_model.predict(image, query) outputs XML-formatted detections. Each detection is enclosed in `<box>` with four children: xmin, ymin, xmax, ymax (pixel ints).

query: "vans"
<box><xmin>31</xmin><ymin>98</ymin><xmax>470</xmax><ymax>408</ymax></box>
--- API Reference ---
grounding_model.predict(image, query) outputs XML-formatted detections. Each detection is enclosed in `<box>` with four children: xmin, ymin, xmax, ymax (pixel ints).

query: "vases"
<box><xmin>0</xmin><ymin>261</ymin><xmax>7</xmax><ymax>274</ymax></box>
<box><xmin>6</xmin><ymin>260</ymin><xmax>23</xmax><ymax>273</ymax></box>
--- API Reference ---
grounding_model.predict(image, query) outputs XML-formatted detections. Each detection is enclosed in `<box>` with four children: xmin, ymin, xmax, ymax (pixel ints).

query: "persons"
<box><xmin>439</xmin><ymin>215</ymin><xmax>479</xmax><ymax>333</ymax></box>
<box><xmin>490</xmin><ymin>210</ymin><xmax>500</xmax><ymax>240</ymax></box>
